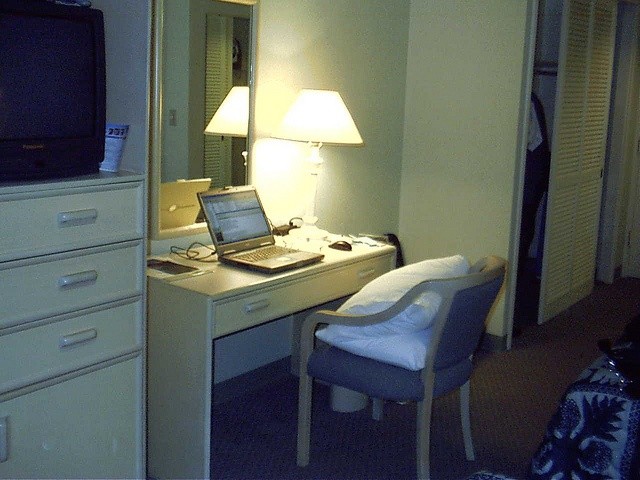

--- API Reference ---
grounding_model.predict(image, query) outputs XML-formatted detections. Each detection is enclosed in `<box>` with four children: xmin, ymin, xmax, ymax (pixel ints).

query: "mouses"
<box><xmin>328</xmin><ymin>241</ymin><xmax>353</xmax><ymax>251</ymax></box>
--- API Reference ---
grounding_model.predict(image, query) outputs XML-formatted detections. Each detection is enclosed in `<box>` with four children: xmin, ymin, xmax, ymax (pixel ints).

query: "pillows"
<box><xmin>328</xmin><ymin>256</ymin><xmax>470</xmax><ymax>339</ymax></box>
<box><xmin>315</xmin><ymin>325</ymin><xmax>432</xmax><ymax>371</ymax></box>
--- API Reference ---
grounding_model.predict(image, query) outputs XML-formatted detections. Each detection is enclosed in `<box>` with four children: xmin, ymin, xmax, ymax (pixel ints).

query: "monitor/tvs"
<box><xmin>0</xmin><ymin>0</ymin><xmax>106</xmax><ymax>187</ymax></box>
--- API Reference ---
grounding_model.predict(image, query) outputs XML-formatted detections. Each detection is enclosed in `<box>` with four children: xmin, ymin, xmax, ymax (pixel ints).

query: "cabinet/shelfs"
<box><xmin>0</xmin><ymin>1</ymin><xmax>149</xmax><ymax>479</ymax></box>
<box><xmin>506</xmin><ymin>1</ymin><xmax>619</xmax><ymax>354</ymax></box>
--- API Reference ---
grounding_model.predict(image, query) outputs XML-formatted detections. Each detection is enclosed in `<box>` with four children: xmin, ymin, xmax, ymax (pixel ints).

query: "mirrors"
<box><xmin>152</xmin><ymin>2</ymin><xmax>258</xmax><ymax>238</ymax></box>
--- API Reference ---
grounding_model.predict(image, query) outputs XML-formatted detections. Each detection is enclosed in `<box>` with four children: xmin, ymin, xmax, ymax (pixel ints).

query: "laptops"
<box><xmin>197</xmin><ymin>185</ymin><xmax>325</xmax><ymax>275</ymax></box>
<box><xmin>159</xmin><ymin>178</ymin><xmax>212</xmax><ymax>231</ymax></box>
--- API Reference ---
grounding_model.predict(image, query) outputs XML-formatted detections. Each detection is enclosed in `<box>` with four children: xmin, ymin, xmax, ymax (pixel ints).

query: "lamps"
<box><xmin>204</xmin><ymin>86</ymin><xmax>250</xmax><ymax>186</ymax></box>
<box><xmin>272</xmin><ymin>88</ymin><xmax>364</xmax><ymax>241</ymax></box>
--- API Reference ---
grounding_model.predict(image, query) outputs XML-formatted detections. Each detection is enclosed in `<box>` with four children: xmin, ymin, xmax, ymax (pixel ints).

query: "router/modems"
<box><xmin>272</xmin><ymin>224</ymin><xmax>299</xmax><ymax>236</ymax></box>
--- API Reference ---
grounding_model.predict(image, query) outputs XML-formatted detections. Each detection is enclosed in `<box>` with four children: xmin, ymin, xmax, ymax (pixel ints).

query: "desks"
<box><xmin>148</xmin><ymin>234</ymin><xmax>397</xmax><ymax>480</ymax></box>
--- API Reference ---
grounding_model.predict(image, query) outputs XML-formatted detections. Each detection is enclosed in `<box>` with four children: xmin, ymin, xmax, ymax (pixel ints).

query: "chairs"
<box><xmin>297</xmin><ymin>252</ymin><xmax>509</xmax><ymax>480</ymax></box>
